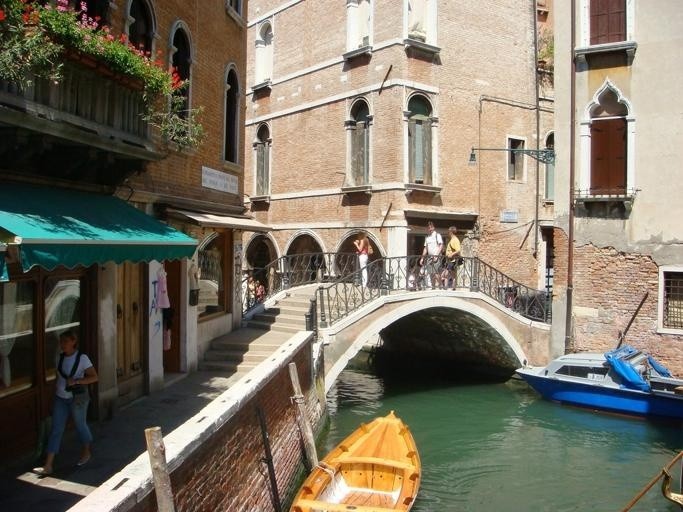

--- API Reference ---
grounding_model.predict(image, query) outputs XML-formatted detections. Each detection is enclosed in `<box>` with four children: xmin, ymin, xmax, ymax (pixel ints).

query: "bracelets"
<box><xmin>76</xmin><ymin>378</ymin><xmax>79</xmax><ymax>384</ymax></box>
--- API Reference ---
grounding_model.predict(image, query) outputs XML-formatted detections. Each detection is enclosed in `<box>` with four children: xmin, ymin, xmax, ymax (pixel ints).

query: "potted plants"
<box><xmin>536</xmin><ymin>28</ymin><xmax>554</xmax><ymax>69</ymax></box>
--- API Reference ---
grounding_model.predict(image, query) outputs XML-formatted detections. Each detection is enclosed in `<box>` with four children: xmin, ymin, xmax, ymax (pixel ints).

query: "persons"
<box><xmin>419</xmin><ymin>222</ymin><xmax>461</xmax><ymax>290</ymax></box>
<box><xmin>255</xmin><ymin>280</ymin><xmax>265</xmax><ymax>303</ymax></box>
<box><xmin>248</xmin><ymin>277</ymin><xmax>256</xmax><ymax>307</ymax></box>
<box><xmin>353</xmin><ymin>230</ymin><xmax>369</xmax><ymax>288</ymax></box>
<box><xmin>33</xmin><ymin>330</ymin><xmax>99</xmax><ymax>475</ymax></box>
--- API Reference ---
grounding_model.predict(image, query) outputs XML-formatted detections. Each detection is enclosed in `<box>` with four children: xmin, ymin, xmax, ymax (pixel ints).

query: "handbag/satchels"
<box><xmin>368</xmin><ymin>245</ymin><xmax>373</xmax><ymax>256</ymax></box>
<box><xmin>459</xmin><ymin>258</ymin><xmax>463</xmax><ymax>265</ymax></box>
<box><xmin>73</xmin><ymin>386</ymin><xmax>90</xmax><ymax>404</ymax></box>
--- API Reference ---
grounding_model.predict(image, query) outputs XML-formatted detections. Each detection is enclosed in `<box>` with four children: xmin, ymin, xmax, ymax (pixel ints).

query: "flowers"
<box><xmin>0</xmin><ymin>0</ymin><xmax>204</xmax><ymax>148</ymax></box>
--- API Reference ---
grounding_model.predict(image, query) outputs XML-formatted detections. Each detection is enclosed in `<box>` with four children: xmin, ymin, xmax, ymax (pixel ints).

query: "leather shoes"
<box><xmin>34</xmin><ymin>464</ymin><xmax>52</xmax><ymax>474</ymax></box>
<box><xmin>76</xmin><ymin>455</ymin><xmax>90</xmax><ymax>465</ymax></box>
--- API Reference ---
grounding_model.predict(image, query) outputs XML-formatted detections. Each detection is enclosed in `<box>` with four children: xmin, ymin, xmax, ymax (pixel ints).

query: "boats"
<box><xmin>289</xmin><ymin>407</ymin><xmax>421</xmax><ymax>512</ymax></box>
<box><xmin>514</xmin><ymin>348</ymin><xmax>683</xmax><ymax>424</ymax></box>
